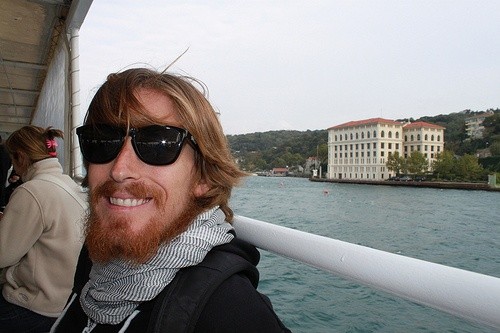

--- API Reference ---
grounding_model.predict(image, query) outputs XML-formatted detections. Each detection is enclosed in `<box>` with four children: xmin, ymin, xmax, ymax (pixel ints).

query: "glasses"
<box><xmin>76</xmin><ymin>121</ymin><xmax>203</xmax><ymax>166</ymax></box>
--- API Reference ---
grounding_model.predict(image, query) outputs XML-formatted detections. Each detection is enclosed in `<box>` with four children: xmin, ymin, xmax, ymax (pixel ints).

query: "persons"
<box><xmin>0</xmin><ymin>125</ymin><xmax>89</xmax><ymax>333</ymax></box>
<box><xmin>51</xmin><ymin>46</ymin><xmax>293</xmax><ymax>333</ymax></box>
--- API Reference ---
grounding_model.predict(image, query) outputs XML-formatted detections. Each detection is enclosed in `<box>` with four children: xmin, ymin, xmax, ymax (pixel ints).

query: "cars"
<box><xmin>386</xmin><ymin>176</ymin><xmax>426</xmax><ymax>182</ymax></box>
